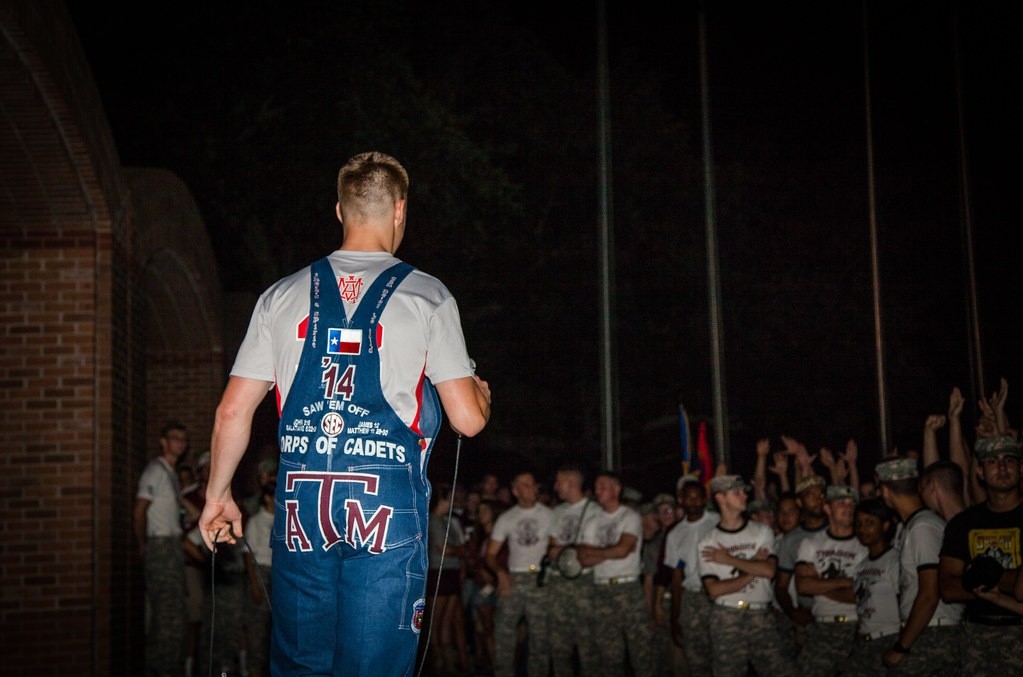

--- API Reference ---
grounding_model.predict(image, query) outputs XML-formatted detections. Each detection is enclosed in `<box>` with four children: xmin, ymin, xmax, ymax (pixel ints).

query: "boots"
<box><xmin>431</xmin><ymin>644</ymin><xmax>462</xmax><ymax>675</ymax></box>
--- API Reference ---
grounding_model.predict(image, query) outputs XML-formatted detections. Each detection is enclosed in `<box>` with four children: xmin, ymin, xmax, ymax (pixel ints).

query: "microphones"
<box><xmin>469</xmin><ymin>358</ymin><xmax>476</xmax><ymax>374</ymax></box>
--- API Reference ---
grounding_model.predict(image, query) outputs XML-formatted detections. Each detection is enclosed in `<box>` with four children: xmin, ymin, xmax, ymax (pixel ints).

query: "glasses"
<box><xmin>166</xmin><ymin>436</ymin><xmax>185</xmax><ymax>444</ymax></box>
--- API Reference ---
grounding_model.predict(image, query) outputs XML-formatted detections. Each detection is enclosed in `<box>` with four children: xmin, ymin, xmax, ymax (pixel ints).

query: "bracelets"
<box><xmin>893</xmin><ymin>641</ymin><xmax>911</xmax><ymax>654</ymax></box>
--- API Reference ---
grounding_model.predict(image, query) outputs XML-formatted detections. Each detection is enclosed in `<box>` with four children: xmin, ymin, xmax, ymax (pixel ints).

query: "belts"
<box><xmin>715</xmin><ymin>598</ymin><xmax>773</xmax><ymax>611</ymax></box>
<box><xmin>147</xmin><ymin>536</ymin><xmax>174</xmax><ymax>543</ymax></box>
<box><xmin>593</xmin><ymin>575</ymin><xmax>637</xmax><ymax>586</ymax></box>
<box><xmin>901</xmin><ymin>618</ymin><xmax>966</xmax><ymax>627</ymax></box>
<box><xmin>508</xmin><ymin>563</ymin><xmax>541</xmax><ymax>573</ymax></box>
<box><xmin>815</xmin><ymin>614</ymin><xmax>858</xmax><ymax>623</ymax></box>
<box><xmin>861</xmin><ymin>623</ymin><xmax>900</xmax><ymax>642</ymax></box>
<box><xmin>552</xmin><ymin>568</ymin><xmax>591</xmax><ymax>576</ymax></box>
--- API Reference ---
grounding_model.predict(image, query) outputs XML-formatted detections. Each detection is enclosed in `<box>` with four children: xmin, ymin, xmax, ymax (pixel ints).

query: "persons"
<box><xmin>131</xmin><ymin>377</ymin><xmax>1023</xmax><ymax>677</ymax></box>
<box><xmin>199</xmin><ymin>152</ymin><xmax>492</xmax><ymax>676</ymax></box>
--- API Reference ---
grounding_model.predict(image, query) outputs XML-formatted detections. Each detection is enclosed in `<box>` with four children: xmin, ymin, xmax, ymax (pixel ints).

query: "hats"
<box><xmin>196</xmin><ymin>450</ymin><xmax>210</xmax><ymax>472</ymax></box>
<box><xmin>826</xmin><ymin>484</ymin><xmax>859</xmax><ymax>505</ymax></box>
<box><xmin>557</xmin><ymin>547</ymin><xmax>582</xmax><ymax>577</ymax></box>
<box><xmin>711</xmin><ymin>475</ymin><xmax>752</xmax><ymax>493</ymax></box>
<box><xmin>748</xmin><ymin>499</ymin><xmax>769</xmax><ymax>513</ymax></box>
<box><xmin>795</xmin><ymin>475</ymin><xmax>826</xmax><ymax>495</ymax></box>
<box><xmin>622</xmin><ymin>487</ymin><xmax>675</xmax><ymax>516</ymax></box>
<box><xmin>874</xmin><ymin>457</ymin><xmax>919</xmax><ymax>482</ymax></box>
<box><xmin>974</xmin><ymin>436</ymin><xmax>1020</xmax><ymax>462</ymax></box>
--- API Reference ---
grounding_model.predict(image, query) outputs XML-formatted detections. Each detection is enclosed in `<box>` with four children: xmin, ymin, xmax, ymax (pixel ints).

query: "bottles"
<box><xmin>535</xmin><ymin>545</ymin><xmax>553</xmax><ymax>585</ymax></box>
